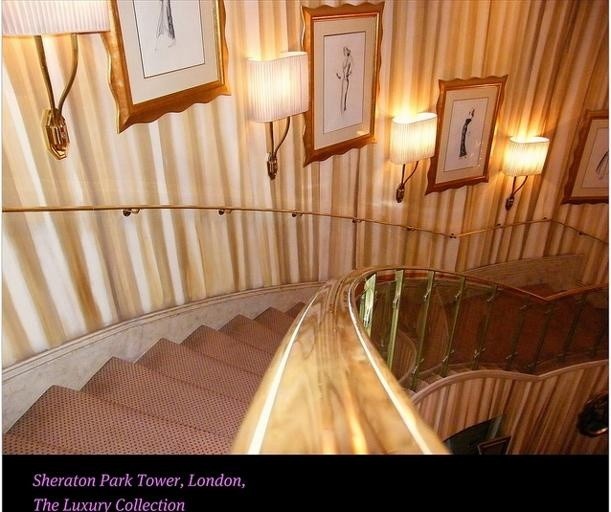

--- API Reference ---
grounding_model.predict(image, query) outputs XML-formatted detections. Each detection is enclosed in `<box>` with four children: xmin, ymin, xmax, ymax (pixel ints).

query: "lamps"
<box><xmin>247</xmin><ymin>51</ymin><xmax>311</xmax><ymax>181</ymax></box>
<box><xmin>3</xmin><ymin>1</ymin><xmax>111</xmax><ymax>159</ymax></box>
<box><xmin>389</xmin><ymin>112</ymin><xmax>439</xmax><ymax>203</ymax></box>
<box><xmin>502</xmin><ymin>136</ymin><xmax>551</xmax><ymax>209</ymax></box>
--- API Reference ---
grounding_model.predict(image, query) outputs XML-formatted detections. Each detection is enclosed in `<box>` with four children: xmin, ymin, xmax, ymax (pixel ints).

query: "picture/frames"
<box><xmin>424</xmin><ymin>75</ymin><xmax>509</xmax><ymax>194</ymax></box>
<box><xmin>561</xmin><ymin>108</ymin><xmax>609</xmax><ymax>204</ymax></box>
<box><xmin>100</xmin><ymin>0</ymin><xmax>234</xmax><ymax>134</ymax></box>
<box><xmin>301</xmin><ymin>1</ymin><xmax>385</xmax><ymax>168</ymax></box>
